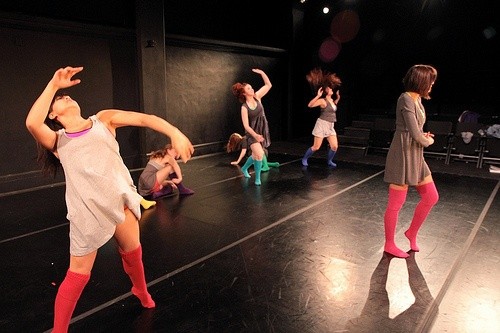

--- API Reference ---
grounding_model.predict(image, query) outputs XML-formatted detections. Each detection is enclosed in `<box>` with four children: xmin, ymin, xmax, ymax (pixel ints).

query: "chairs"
<box><xmin>338</xmin><ymin>119</ymin><xmax>500</xmax><ymax>168</ymax></box>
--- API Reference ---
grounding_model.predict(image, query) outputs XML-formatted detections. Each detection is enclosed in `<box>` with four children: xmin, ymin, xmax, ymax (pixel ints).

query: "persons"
<box><xmin>302</xmin><ymin>69</ymin><xmax>341</xmax><ymax>167</ymax></box>
<box><xmin>382</xmin><ymin>65</ymin><xmax>440</xmax><ymax>257</ymax></box>
<box><xmin>25</xmin><ymin>66</ymin><xmax>194</xmax><ymax>333</ymax></box>
<box><xmin>231</xmin><ymin>68</ymin><xmax>273</xmax><ymax>186</ymax></box>
<box><xmin>137</xmin><ymin>141</ymin><xmax>195</xmax><ymax>199</ymax></box>
<box><xmin>226</xmin><ymin>133</ymin><xmax>280</xmax><ymax>172</ymax></box>
